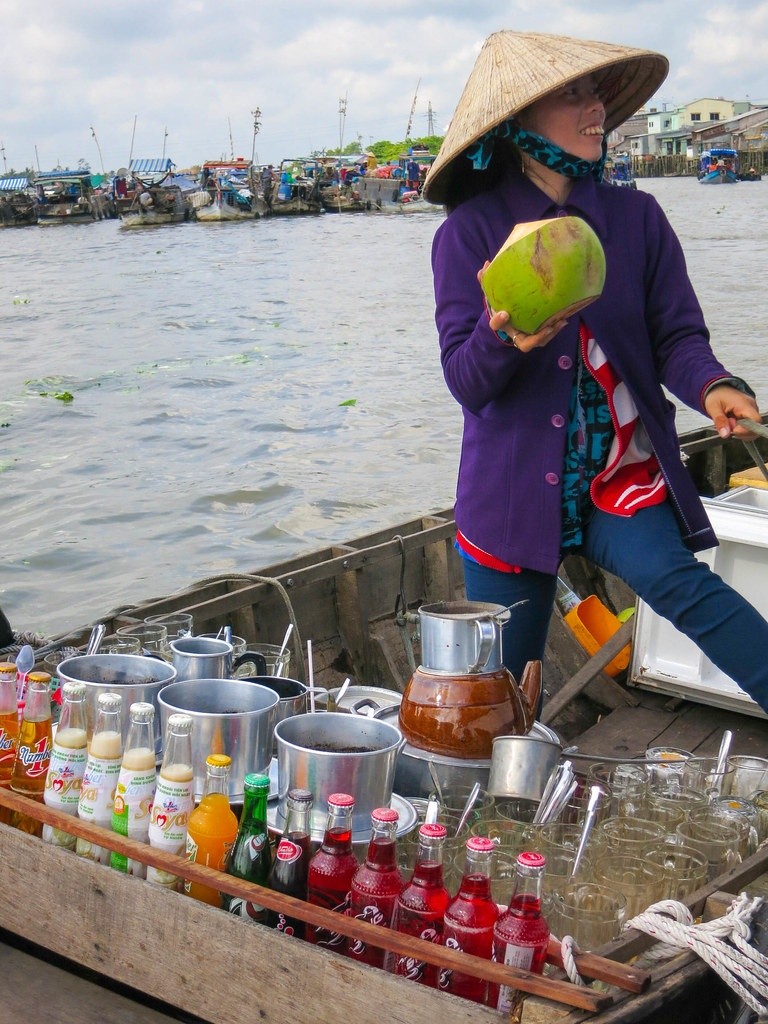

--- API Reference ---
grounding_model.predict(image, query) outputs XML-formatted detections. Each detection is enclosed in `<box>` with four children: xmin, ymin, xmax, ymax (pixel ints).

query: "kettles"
<box><xmin>397</xmin><ymin>660</ymin><xmax>543</xmax><ymax>760</ymax></box>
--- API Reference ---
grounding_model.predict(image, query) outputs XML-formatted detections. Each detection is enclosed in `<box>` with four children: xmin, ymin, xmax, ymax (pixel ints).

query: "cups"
<box><xmin>393</xmin><ymin>736</ymin><xmax>768</xmax><ymax>951</ymax></box>
<box><xmin>43</xmin><ymin>612</ymin><xmax>291</xmax><ymax>679</ymax></box>
<box><xmin>418</xmin><ymin>601</ymin><xmax>512</xmax><ymax>674</ymax></box>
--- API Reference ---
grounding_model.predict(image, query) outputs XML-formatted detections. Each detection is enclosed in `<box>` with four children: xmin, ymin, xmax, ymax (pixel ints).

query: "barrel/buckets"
<box><xmin>169</xmin><ymin>635</ymin><xmax>233</xmax><ymax>683</ymax></box>
<box><xmin>157</xmin><ymin>678</ymin><xmax>281</xmax><ymax>795</ymax></box>
<box><xmin>274</xmin><ymin>712</ymin><xmax>407</xmax><ymax>840</ymax></box>
<box><xmin>55</xmin><ymin>653</ymin><xmax>177</xmax><ymax>765</ymax></box>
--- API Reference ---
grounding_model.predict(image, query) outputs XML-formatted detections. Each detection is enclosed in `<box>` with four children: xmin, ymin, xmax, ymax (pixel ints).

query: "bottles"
<box><xmin>0</xmin><ymin>660</ymin><xmax>550</xmax><ymax>1015</ymax></box>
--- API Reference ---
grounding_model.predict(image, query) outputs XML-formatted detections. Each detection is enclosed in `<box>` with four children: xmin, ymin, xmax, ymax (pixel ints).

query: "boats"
<box><xmin>697</xmin><ymin>149</ymin><xmax>738</xmax><ymax>184</ymax></box>
<box><xmin>604</xmin><ymin>157</ymin><xmax>637</xmax><ymax>189</ymax></box>
<box><xmin>0</xmin><ymin>144</ymin><xmax>444</xmax><ymax>226</ymax></box>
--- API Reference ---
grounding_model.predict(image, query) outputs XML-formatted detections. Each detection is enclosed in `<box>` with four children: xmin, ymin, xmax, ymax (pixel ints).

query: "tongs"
<box><xmin>736</xmin><ymin>418</ymin><xmax>768</xmax><ymax>481</ymax></box>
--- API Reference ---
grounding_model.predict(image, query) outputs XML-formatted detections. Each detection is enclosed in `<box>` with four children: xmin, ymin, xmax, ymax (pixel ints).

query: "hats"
<box><xmin>267</xmin><ymin>165</ymin><xmax>275</xmax><ymax>168</ymax></box>
<box><xmin>421</xmin><ymin>29</ymin><xmax>671</xmax><ymax>207</ymax></box>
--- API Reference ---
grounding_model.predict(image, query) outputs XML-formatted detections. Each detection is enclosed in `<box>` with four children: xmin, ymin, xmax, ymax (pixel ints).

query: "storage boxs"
<box><xmin>728</xmin><ymin>460</ymin><xmax>768</xmax><ymax>489</ymax></box>
<box><xmin>628</xmin><ymin>484</ymin><xmax>768</xmax><ymax>719</ymax></box>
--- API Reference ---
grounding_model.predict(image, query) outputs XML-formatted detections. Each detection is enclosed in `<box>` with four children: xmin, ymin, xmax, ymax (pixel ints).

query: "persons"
<box><xmin>711</xmin><ymin>156</ymin><xmax>732</xmax><ymax>170</ymax></box>
<box><xmin>418</xmin><ymin>168</ymin><xmax>427</xmax><ymax>193</ymax></box>
<box><xmin>353</xmin><ymin>161</ymin><xmax>393</xmax><ymax>175</ymax></box>
<box><xmin>421</xmin><ymin>30</ymin><xmax>768</xmax><ymax>721</ymax></box>
<box><xmin>263</xmin><ymin>165</ymin><xmax>277</xmax><ymax>199</ymax></box>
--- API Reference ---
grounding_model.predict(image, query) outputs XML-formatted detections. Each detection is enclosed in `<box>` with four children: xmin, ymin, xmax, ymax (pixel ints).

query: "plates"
<box><xmin>193</xmin><ymin>758</ymin><xmax>279</xmax><ymax>806</ymax></box>
<box><xmin>252</xmin><ymin>792</ymin><xmax>419</xmax><ymax>846</ymax></box>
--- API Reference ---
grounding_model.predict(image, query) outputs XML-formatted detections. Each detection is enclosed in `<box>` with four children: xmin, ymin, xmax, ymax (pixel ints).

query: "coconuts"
<box><xmin>481</xmin><ymin>216</ymin><xmax>606</xmax><ymax>336</ymax></box>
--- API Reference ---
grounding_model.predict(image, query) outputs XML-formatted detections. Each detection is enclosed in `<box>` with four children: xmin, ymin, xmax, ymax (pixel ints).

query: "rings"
<box><xmin>513</xmin><ymin>332</ymin><xmax>522</xmax><ymax>349</ymax></box>
